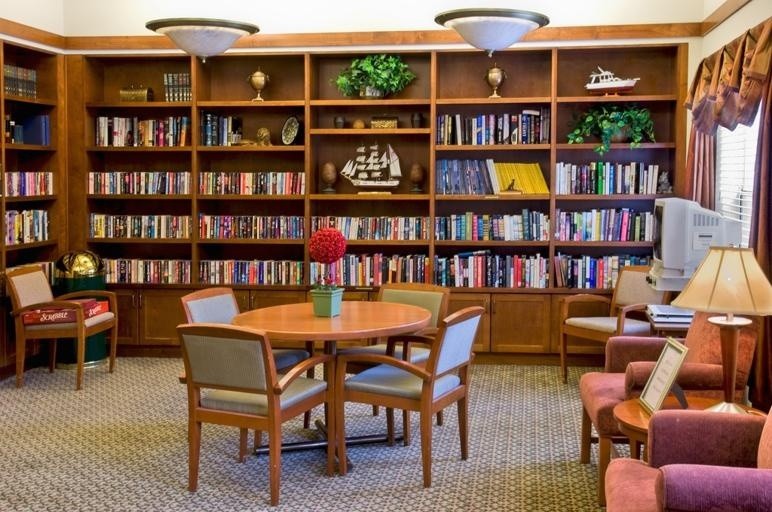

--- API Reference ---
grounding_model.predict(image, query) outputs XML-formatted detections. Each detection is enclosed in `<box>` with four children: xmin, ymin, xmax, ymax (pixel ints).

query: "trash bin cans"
<box><xmin>53</xmin><ymin>252</ymin><xmax>110</xmax><ymax>372</ymax></box>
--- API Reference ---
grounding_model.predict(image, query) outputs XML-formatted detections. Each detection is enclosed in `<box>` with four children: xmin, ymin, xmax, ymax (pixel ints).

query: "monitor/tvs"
<box><xmin>651</xmin><ymin>197</ymin><xmax>743</xmax><ymax>278</ymax></box>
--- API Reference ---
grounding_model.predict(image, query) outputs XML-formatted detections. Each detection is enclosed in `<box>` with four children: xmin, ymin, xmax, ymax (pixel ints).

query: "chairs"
<box><xmin>181</xmin><ymin>286</ymin><xmax>311</xmax><ymax>454</ymax></box>
<box><xmin>335</xmin><ymin>283</ymin><xmax>452</xmax><ymax>447</ymax></box>
<box><xmin>174</xmin><ymin>323</ymin><xmax>336</xmax><ymax>509</ymax></box>
<box><xmin>578</xmin><ymin>310</ymin><xmax>760</xmax><ymax>506</ymax></box>
<box><xmin>558</xmin><ymin>265</ymin><xmax>674</xmax><ymax>385</ymax></box>
<box><xmin>2</xmin><ymin>264</ymin><xmax>120</xmax><ymax>391</ymax></box>
<box><xmin>604</xmin><ymin>406</ymin><xmax>772</xmax><ymax>512</ymax></box>
<box><xmin>337</xmin><ymin>306</ymin><xmax>487</xmax><ymax>489</ymax></box>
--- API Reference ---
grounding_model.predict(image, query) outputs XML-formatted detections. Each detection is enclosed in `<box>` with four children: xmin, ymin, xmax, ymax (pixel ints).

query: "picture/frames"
<box><xmin>637</xmin><ymin>338</ymin><xmax>690</xmax><ymax>417</ymax></box>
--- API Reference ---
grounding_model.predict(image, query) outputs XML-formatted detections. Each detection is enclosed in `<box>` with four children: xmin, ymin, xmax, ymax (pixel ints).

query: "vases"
<box><xmin>309</xmin><ymin>287</ymin><xmax>342</xmax><ymax>318</ymax></box>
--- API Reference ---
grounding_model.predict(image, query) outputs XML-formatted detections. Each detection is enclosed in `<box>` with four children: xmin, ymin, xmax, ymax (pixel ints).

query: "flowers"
<box><xmin>307</xmin><ymin>226</ymin><xmax>347</xmax><ymax>290</ymax></box>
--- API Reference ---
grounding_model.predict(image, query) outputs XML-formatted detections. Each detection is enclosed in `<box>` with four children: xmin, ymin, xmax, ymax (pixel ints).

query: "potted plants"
<box><xmin>327</xmin><ymin>54</ymin><xmax>418</xmax><ymax>99</ymax></box>
<box><xmin>566</xmin><ymin>106</ymin><xmax>658</xmax><ymax>157</ymax></box>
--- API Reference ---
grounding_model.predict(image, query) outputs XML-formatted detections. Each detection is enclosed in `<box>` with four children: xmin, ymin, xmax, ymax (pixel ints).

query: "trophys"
<box><xmin>245</xmin><ymin>65</ymin><xmax>272</xmax><ymax>102</ymax></box>
<box><xmin>483</xmin><ymin>61</ymin><xmax>507</xmax><ymax>98</ymax></box>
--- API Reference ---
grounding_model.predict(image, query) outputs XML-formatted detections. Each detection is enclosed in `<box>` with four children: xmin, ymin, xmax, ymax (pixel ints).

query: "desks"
<box><xmin>230</xmin><ymin>299</ymin><xmax>435</xmax><ymax>471</ymax></box>
<box><xmin>611</xmin><ymin>394</ymin><xmax>764</xmax><ymax>460</ymax></box>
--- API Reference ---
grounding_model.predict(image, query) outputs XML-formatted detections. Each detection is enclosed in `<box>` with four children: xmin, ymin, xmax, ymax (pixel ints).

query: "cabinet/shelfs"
<box><xmin>553</xmin><ymin>42</ymin><xmax>689</xmax><ymax>355</ymax></box>
<box><xmin>0</xmin><ymin>34</ymin><xmax>66</xmax><ymax>379</ymax></box>
<box><xmin>307</xmin><ymin>47</ymin><xmax>434</xmax><ymax>352</ymax></box>
<box><xmin>68</xmin><ymin>51</ymin><xmax>194</xmax><ymax>346</ymax></box>
<box><xmin>194</xmin><ymin>50</ymin><xmax>307</xmax><ymax>312</ymax></box>
<box><xmin>436</xmin><ymin>46</ymin><xmax>554</xmax><ymax>354</ymax></box>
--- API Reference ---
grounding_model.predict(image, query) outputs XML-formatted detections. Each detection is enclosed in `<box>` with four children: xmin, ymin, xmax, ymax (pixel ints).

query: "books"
<box><xmin>646</xmin><ymin>303</ymin><xmax>695</xmax><ymax>323</ymax></box>
<box><xmin>163</xmin><ymin>72</ymin><xmax>192</xmax><ymax>102</ymax></box>
<box><xmin>4</xmin><ymin>62</ymin><xmax>53</xmax><ymax>245</ymax></box>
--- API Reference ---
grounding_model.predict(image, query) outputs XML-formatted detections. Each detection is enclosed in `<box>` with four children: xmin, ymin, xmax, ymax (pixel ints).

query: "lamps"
<box><xmin>673</xmin><ymin>243</ymin><xmax>772</xmax><ymax>414</ymax></box>
<box><xmin>433</xmin><ymin>5</ymin><xmax>551</xmax><ymax>57</ymax></box>
<box><xmin>146</xmin><ymin>15</ymin><xmax>261</xmax><ymax>65</ymax></box>
